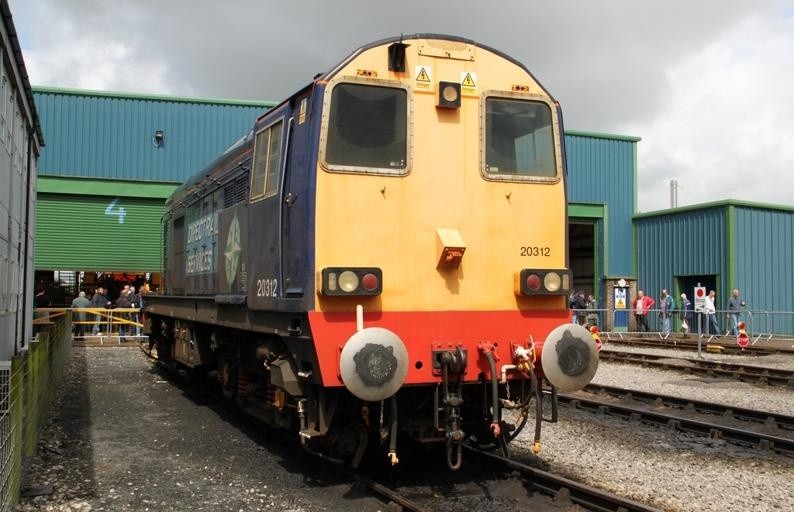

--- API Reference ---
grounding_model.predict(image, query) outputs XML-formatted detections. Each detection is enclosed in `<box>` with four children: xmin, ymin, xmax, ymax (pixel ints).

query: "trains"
<box><xmin>141</xmin><ymin>33</ymin><xmax>599</xmax><ymax>487</ymax></box>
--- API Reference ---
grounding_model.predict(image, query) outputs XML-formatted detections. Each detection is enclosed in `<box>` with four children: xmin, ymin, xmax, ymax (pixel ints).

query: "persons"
<box><xmin>70</xmin><ymin>291</ymin><xmax>93</xmax><ymax>340</ymax></box>
<box><xmin>725</xmin><ymin>288</ymin><xmax>746</xmax><ymax>335</ymax></box>
<box><xmin>704</xmin><ymin>290</ymin><xmax>721</xmax><ymax>338</ymax></box>
<box><xmin>91</xmin><ymin>285</ymin><xmax>152</xmax><ymax>343</ymax></box>
<box><xmin>678</xmin><ymin>294</ymin><xmax>693</xmax><ymax>339</ymax></box>
<box><xmin>33</xmin><ymin>286</ymin><xmax>51</xmax><ymax>338</ymax></box>
<box><xmin>632</xmin><ymin>290</ymin><xmax>655</xmax><ymax>331</ymax></box>
<box><xmin>615</xmin><ymin>288</ymin><xmax>625</xmax><ymax>298</ymax></box>
<box><xmin>567</xmin><ymin>290</ymin><xmax>600</xmax><ymax>333</ymax></box>
<box><xmin>656</xmin><ymin>287</ymin><xmax>675</xmax><ymax>333</ymax></box>
